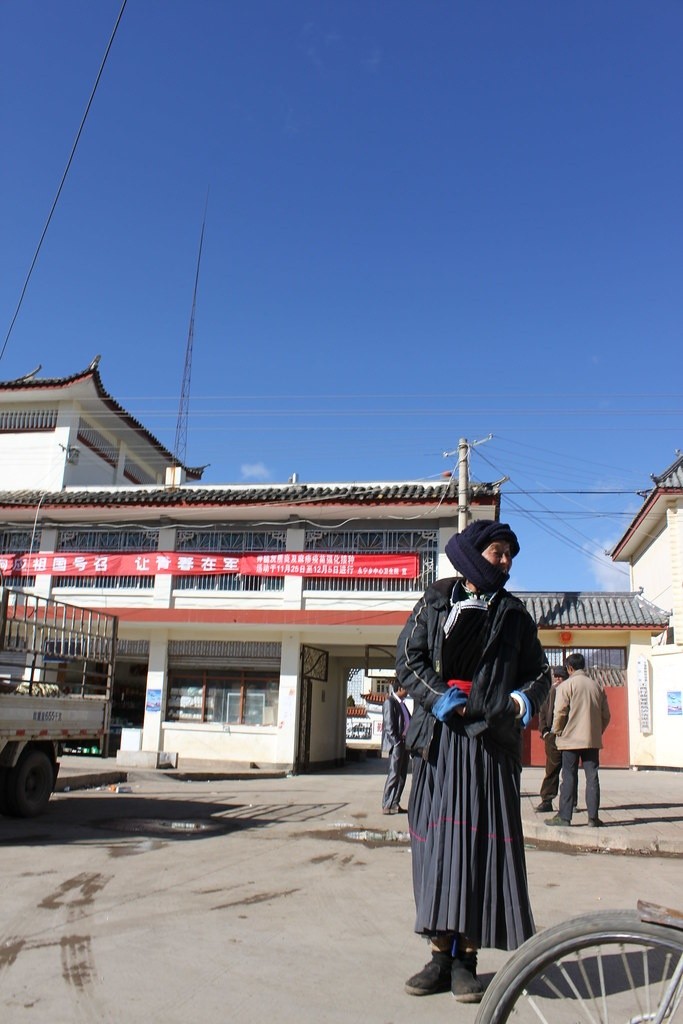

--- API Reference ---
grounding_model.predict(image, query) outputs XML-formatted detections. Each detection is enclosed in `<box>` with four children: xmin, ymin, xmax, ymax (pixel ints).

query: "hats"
<box><xmin>552</xmin><ymin>666</ymin><xmax>568</xmax><ymax>677</ymax></box>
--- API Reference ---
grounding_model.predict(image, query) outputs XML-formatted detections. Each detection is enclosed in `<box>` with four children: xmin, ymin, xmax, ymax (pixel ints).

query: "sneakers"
<box><xmin>588</xmin><ymin>818</ymin><xmax>603</xmax><ymax>827</ymax></box>
<box><xmin>544</xmin><ymin>813</ymin><xmax>570</xmax><ymax>826</ymax></box>
<box><xmin>534</xmin><ymin>801</ymin><xmax>553</xmax><ymax>811</ymax></box>
<box><xmin>573</xmin><ymin>806</ymin><xmax>577</xmax><ymax>812</ymax></box>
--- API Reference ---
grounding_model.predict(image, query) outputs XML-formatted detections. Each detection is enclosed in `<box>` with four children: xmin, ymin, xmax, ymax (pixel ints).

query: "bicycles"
<box><xmin>474</xmin><ymin>899</ymin><xmax>683</xmax><ymax>1024</ymax></box>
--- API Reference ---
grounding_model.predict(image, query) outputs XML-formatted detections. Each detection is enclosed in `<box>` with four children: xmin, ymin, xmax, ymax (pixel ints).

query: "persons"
<box><xmin>381</xmin><ymin>681</ymin><xmax>412</xmax><ymax>814</ymax></box>
<box><xmin>533</xmin><ymin>665</ymin><xmax>579</xmax><ymax>813</ymax></box>
<box><xmin>544</xmin><ymin>653</ymin><xmax>611</xmax><ymax>827</ymax></box>
<box><xmin>397</xmin><ymin>519</ymin><xmax>553</xmax><ymax>1002</ymax></box>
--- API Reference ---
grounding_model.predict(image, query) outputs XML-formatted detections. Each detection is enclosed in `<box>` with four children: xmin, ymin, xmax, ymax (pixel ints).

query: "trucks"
<box><xmin>0</xmin><ymin>590</ymin><xmax>119</xmax><ymax>818</ymax></box>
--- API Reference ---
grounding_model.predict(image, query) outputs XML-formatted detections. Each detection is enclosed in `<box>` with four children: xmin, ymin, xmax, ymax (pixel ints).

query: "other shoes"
<box><xmin>450</xmin><ymin>957</ymin><xmax>486</xmax><ymax>1001</ymax></box>
<box><xmin>404</xmin><ymin>951</ymin><xmax>451</xmax><ymax>994</ymax></box>
<box><xmin>382</xmin><ymin>804</ymin><xmax>407</xmax><ymax>814</ymax></box>
